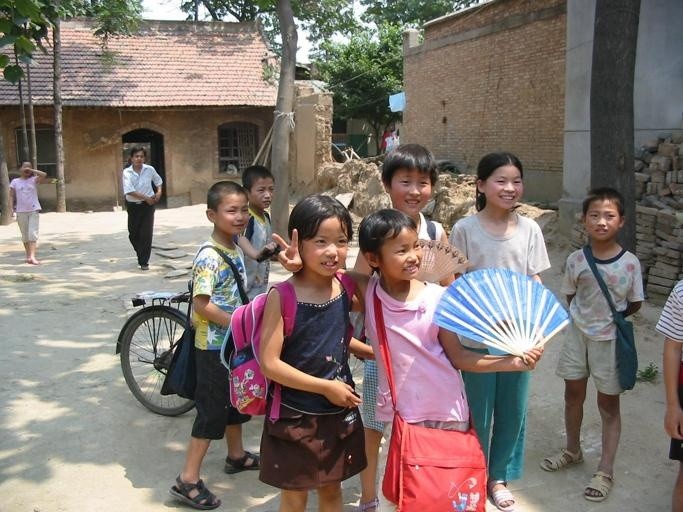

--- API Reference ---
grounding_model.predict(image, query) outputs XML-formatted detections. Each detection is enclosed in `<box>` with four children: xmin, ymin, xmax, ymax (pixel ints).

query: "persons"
<box><xmin>6</xmin><ymin>159</ymin><xmax>48</xmax><ymax>266</ymax></box>
<box><xmin>539</xmin><ymin>188</ymin><xmax>645</xmax><ymax>503</ymax></box>
<box><xmin>122</xmin><ymin>146</ymin><xmax>165</xmax><ymax>271</ymax></box>
<box><xmin>447</xmin><ymin>151</ymin><xmax>551</xmax><ymax>511</ymax></box>
<box><xmin>655</xmin><ymin>276</ymin><xmax>683</xmax><ymax>511</ymax></box>
<box><xmin>269</xmin><ymin>209</ymin><xmax>545</xmax><ymax>511</ymax></box>
<box><xmin>255</xmin><ymin>194</ymin><xmax>375</xmax><ymax>510</ymax></box>
<box><xmin>350</xmin><ymin>145</ymin><xmax>458</xmax><ymax>511</ymax></box>
<box><xmin>168</xmin><ymin>179</ymin><xmax>261</xmax><ymax>510</ymax></box>
<box><xmin>230</xmin><ymin>163</ymin><xmax>279</xmax><ymax>304</ymax></box>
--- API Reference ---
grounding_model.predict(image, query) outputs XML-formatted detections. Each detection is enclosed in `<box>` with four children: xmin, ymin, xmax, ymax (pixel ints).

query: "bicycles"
<box><xmin>116</xmin><ymin>274</ymin><xmax>367</xmax><ymax>417</ymax></box>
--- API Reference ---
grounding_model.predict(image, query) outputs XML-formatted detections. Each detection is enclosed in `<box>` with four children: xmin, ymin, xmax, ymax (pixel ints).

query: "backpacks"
<box><xmin>220</xmin><ymin>271</ymin><xmax>356</xmax><ymax>416</ymax></box>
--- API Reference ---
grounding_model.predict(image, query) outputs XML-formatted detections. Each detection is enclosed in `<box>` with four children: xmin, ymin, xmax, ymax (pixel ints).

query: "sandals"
<box><xmin>357</xmin><ymin>496</ymin><xmax>379</xmax><ymax>512</ymax></box>
<box><xmin>487</xmin><ymin>478</ymin><xmax>517</xmax><ymax>512</ymax></box>
<box><xmin>539</xmin><ymin>448</ymin><xmax>585</xmax><ymax>472</ymax></box>
<box><xmin>584</xmin><ymin>470</ymin><xmax>617</xmax><ymax>502</ymax></box>
<box><xmin>224</xmin><ymin>449</ymin><xmax>260</xmax><ymax>474</ymax></box>
<box><xmin>168</xmin><ymin>474</ymin><xmax>221</xmax><ymax>510</ymax></box>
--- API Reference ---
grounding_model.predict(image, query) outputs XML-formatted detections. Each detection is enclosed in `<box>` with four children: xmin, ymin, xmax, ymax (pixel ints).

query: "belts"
<box><xmin>127</xmin><ymin>200</ymin><xmax>145</xmax><ymax>206</ymax></box>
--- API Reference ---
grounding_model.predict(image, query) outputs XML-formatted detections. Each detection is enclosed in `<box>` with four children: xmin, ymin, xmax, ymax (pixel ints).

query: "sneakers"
<box><xmin>137</xmin><ymin>255</ymin><xmax>150</xmax><ymax>270</ymax></box>
<box><xmin>25</xmin><ymin>256</ymin><xmax>40</xmax><ymax>265</ymax></box>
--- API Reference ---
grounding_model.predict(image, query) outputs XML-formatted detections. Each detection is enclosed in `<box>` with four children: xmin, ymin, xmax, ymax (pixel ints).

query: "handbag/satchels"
<box><xmin>612</xmin><ymin>312</ymin><xmax>638</xmax><ymax>391</ymax></box>
<box><xmin>160</xmin><ymin>327</ymin><xmax>197</xmax><ymax>400</ymax></box>
<box><xmin>381</xmin><ymin>409</ymin><xmax>487</xmax><ymax>512</ymax></box>
